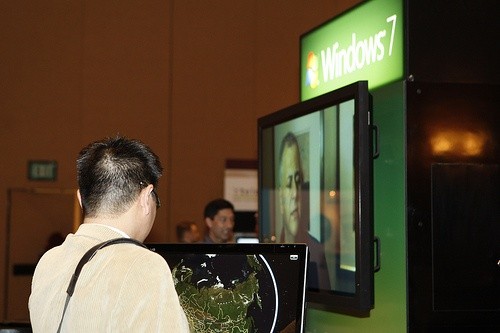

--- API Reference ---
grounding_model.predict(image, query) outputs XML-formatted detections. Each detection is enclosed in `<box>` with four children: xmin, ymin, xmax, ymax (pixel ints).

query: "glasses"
<box><xmin>139</xmin><ymin>181</ymin><xmax>160</xmax><ymax>209</ymax></box>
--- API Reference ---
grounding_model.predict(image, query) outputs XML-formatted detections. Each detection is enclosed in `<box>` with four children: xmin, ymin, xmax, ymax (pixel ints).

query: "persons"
<box><xmin>277</xmin><ymin>134</ymin><xmax>332</xmax><ymax>289</ymax></box>
<box><xmin>202</xmin><ymin>198</ymin><xmax>235</xmax><ymax>243</ymax></box>
<box><xmin>27</xmin><ymin>136</ymin><xmax>189</xmax><ymax>333</ymax></box>
<box><xmin>176</xmin><ymin>222</ymin><xmax>199</xmax><ymax>243</ymax></box>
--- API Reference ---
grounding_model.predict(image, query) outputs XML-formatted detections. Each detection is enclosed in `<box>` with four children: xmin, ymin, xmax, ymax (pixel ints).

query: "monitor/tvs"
<box><xmin>254</xmin><ymin>82</ymin><xmax>375</xmax><ymax>318</ymax></box>
<box><xmin>146</xmin><ymin>242</ymin><xmax>309</xmax><ymax>333</ymax></box>
